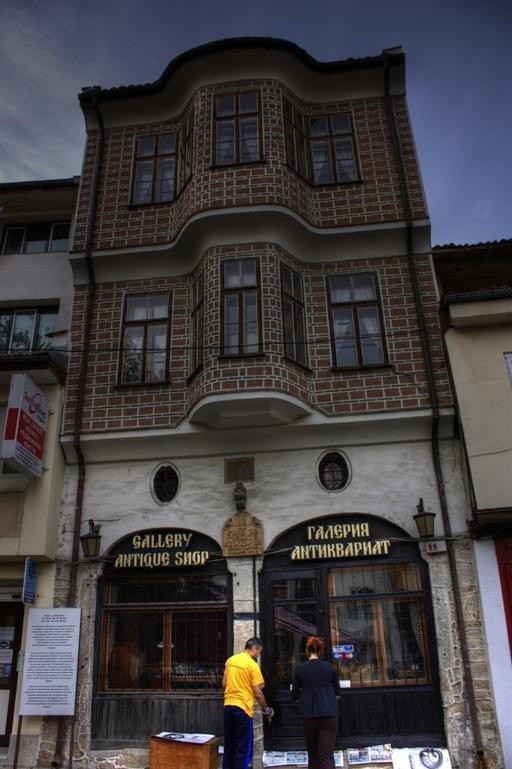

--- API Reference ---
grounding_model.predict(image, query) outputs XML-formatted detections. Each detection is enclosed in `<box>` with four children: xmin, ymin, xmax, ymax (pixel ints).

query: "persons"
<box><xmin>233</xmin><ymin>480</ymin><xmax>247</xmax><ymax>509</ymax></box>
<box><xmin>222</xmin><ymin>637</ymin><xmax>275</xmax><ymax>769</ymax></box>
<box><xmin>291</xmin><ymin>636</ymin><xmax>341</xmax><ymax>769</ymax></box>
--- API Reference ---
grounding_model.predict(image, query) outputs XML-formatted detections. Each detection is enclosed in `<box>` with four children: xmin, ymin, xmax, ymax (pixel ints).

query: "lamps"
<box><xmin>415</xmin><ymin>494</ymin><xmax>436</xmax><ymax>539</ymax></box>
<box><xmin>79</xmin><ymin>517</ymin><xmax>104</xmax><ymax>557</ymax></box>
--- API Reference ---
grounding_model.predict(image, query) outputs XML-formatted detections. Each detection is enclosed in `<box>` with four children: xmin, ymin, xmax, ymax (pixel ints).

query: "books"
<box><xmin>262</xmin><ymin>744</ymin><xmax>452</xmax><ymax>769</ymax></box>
